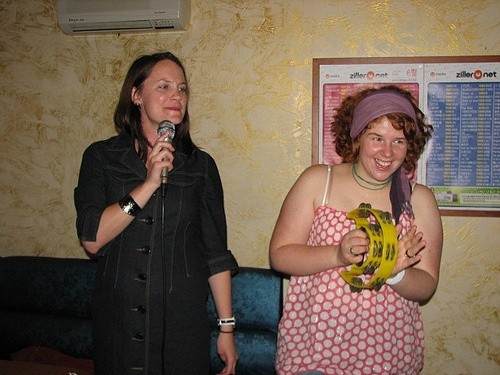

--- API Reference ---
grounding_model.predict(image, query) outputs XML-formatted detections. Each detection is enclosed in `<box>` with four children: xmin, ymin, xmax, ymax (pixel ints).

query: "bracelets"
<box><xmin>219</xmin><ymin>330</ymin><xmax>234</xmax><ymax>334</ymax></box>
<box><xmin>386</xmin><ymin>270</ymin><xmax>405</xmax><ymax>285</ymax></box>
<box><xmin>119</xmin><ymin>195</ymin><xmax>142</xmax><ymax>217</ymax></box>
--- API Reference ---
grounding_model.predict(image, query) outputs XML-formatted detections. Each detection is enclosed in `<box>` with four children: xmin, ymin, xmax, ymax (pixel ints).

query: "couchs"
<box><xmin>0</xmin><ymin>256</ymin><xmax>283</xmax><ymax>375</ymax></box>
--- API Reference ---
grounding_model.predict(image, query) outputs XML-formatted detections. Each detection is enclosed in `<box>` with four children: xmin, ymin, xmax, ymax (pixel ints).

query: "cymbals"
<box><xmin>339</xmin><ymin>203</ymin><xmax>398</xmax><ymax>294</ymax></box>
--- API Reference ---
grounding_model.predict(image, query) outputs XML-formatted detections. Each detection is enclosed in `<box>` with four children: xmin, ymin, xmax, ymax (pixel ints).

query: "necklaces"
<box><xmin>352</xmin><ymin>161</ymin><xmax>392</xmax><ymax>190</ymax></box>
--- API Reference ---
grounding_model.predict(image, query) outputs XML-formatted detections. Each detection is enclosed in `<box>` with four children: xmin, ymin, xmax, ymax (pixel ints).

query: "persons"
<box><xmin>74</xmin><ymin>52</ymin><xmax>239</xmax><ymax>375</ymax></box>
<box><xmin>269</xmin><ymin>85</ymin><xmax>444</xmax><ymax>375</ymax></box>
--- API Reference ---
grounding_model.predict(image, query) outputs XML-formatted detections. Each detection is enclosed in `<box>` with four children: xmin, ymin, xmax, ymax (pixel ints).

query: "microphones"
<box><xmin>157</xmin><ymin>120</ymin><xmax>176</xmax><ymax>197</ymax></box>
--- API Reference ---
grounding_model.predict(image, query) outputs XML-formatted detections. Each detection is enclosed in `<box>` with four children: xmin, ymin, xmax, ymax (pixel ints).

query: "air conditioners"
<box><xmin>57</xmin><ymin>0</ymin><xmax>191</xmax><ymax>36</ymax></box>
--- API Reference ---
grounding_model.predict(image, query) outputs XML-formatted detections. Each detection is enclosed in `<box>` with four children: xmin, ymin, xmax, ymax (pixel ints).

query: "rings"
<box><xmin>406</xmin><ymin>248</ymin><xmax>415</xmax><ymax>258</ymax></box>
<box><xmin>350</xmin><ymin>247</ymin><xmax>354</xmax><ymax>254</ymax></box>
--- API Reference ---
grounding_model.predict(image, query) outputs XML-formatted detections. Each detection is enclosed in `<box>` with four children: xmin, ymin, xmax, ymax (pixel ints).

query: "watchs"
<box><xmin>217</xmin><ymin>316</ymin><xmax>235</xmax><ymax>326</ymax></box>
